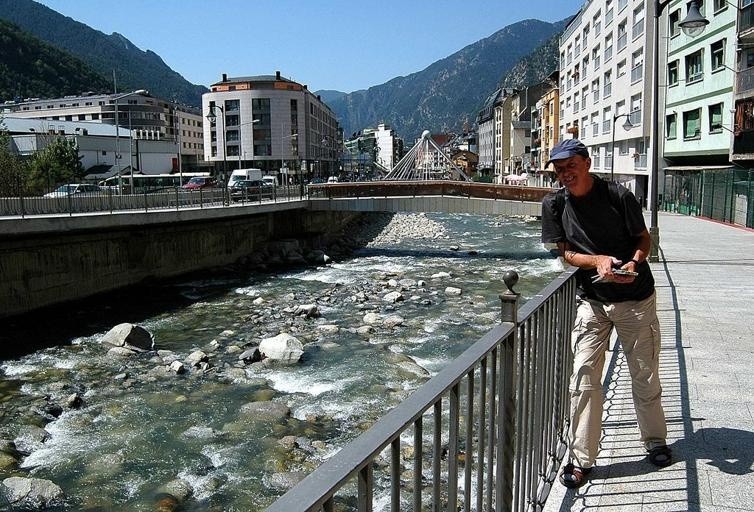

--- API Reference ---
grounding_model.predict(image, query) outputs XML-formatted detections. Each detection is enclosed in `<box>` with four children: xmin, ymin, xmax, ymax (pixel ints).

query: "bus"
<box><xmin>98</xmin><ymin>172</ymin><xmax>210</xmax><ymax>190</ymax></box>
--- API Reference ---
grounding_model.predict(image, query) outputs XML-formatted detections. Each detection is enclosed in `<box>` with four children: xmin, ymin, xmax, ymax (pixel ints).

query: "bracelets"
<box><xmin>627</xmin><ymin>259</ymin><xmax>638</xmax><ymax>268</ymax></box>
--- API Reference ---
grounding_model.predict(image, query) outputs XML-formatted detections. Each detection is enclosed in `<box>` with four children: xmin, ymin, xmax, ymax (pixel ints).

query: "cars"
<box><xmin>181</xmin><ymin>176</ymin><xmax>217</xmax><ymax>191</ymax></box>
<box><xmin>230</xmin><ymin>180</ymin><xmax>276</xmax><ymax>202</ymax></box>
<box><xmin>260</xmin><ymin>176</ymin><xmax>279</xmax><ymax>188</ymax></box>
<box><xmin>43</xmin><ymin>177</ymin><xmax>100</xmax><ymax>197</ymax></box>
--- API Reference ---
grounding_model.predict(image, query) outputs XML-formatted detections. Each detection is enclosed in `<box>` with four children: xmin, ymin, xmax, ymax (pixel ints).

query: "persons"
<box><xmin>542</xmin><ymin>139</ymin><xmax>671</xmax><ymax>488</ymax></box>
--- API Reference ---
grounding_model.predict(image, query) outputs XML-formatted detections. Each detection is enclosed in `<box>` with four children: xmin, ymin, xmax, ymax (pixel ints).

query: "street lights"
<box><xmin>112</xmin><ymin>68</ymin><xmax>134</xmax><ymax>195</ymax></box>
<box><xmin>238</xmin><ymin>106</ymin><xmax>260</xmax><ymax>169</ymax></box>
<box><xmin>649</xmin><ymin>0</ymin><xmax>710</xmax><ymax>263</ymax></box>
<box><xmin>205</xmin><ymin>100</ymin><xmax>229</xmax><ymax>206</ymax></box>
<box><xmin>611</xmin><ymin>112</ymin><xmax>633</xmax><ymax>180</ymax></box>
<box><xmin>281</xmin><ymin>122</ymin><xmax>299</xmax><ymax>186</ymax></box>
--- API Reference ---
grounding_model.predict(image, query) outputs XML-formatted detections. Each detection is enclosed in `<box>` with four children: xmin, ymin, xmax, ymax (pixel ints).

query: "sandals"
<box><xmin>559</xmin><ymin>463</ymin><xmax>591</xmax><ymax>488</ymax></box>
<box><xmin>647</xmin><ymin>446</ymin><xmax>672</xmax><ymax>467</ymax></box>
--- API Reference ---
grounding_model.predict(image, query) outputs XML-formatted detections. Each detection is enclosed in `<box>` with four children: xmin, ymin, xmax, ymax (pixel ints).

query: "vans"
<box><xmin>227</xmin><ymin>169</ymin><xmax>262</xmax><ymax>191</ymax></box>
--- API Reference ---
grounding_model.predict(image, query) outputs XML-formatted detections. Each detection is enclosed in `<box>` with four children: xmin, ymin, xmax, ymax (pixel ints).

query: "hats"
<box><xmin>545</xmin><ymin>139</ymin><xmax>587</xmax><ymax>169</ymax></box>
